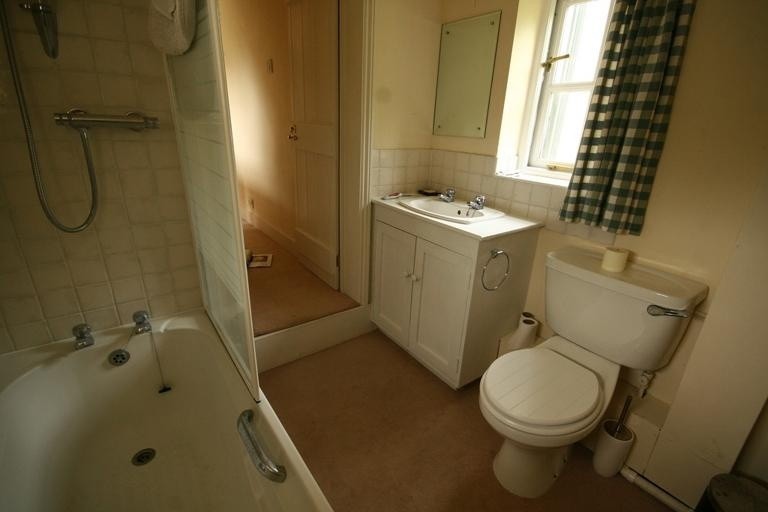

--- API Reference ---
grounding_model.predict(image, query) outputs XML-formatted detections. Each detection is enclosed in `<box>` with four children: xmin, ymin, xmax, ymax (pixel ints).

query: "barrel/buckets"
<box><xmin>692</xmin><ymin>472</ymin><xmax>768</xmax><ymax>512</ymax></box>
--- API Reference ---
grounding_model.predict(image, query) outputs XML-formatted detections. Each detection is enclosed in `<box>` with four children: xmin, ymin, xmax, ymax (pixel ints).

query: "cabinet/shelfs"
<box><xmin>371</xmin><ymin>203</ymin><xmax>541</xmax><ymax>392</ymax></box>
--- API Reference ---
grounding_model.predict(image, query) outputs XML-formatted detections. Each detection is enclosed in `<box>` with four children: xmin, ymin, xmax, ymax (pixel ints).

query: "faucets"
<box><xmin>438</xmin><ymin>190</ymin><xmax>456</xmax><ymax>201</ymax></box>
<box><xmin>467</xmin><ymin>195</ymin><xmax>485</xmax><ymax>210</ymax></box>
<box><xmin>71</xmin><ymin>324</ymin><xmax>95</xmax><ymax>350</ymax></box>
<box><xmin>132</xmin><ymin>311</ymin><xmax>151</xmax><ymax>334</ymax></box>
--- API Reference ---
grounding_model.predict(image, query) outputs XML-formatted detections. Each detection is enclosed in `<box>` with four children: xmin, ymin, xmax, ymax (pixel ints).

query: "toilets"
<box><xmin>479</xmin><ymin>246</ymin><xmax>709</xmax><ymax>498</ymax></box>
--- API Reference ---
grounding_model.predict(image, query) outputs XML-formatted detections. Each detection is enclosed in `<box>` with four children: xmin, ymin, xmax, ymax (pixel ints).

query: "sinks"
<box><xmin>399</xmin><ymin>196</ymin><xmax>505</xmax><ymax>224</ymax></box>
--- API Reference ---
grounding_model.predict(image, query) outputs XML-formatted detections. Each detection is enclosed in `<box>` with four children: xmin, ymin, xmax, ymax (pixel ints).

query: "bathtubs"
<box><xmin>0</xmin><ymin>311</ymin><xmax>334</xmax><ymax>512</ymax></box>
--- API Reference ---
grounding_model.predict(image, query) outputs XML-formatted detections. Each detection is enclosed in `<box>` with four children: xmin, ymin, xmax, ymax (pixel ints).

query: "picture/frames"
<box><xmin>434</xmin><ymin>12</ymin><xmax>501</xmax><ymax>136</ymax></box>
